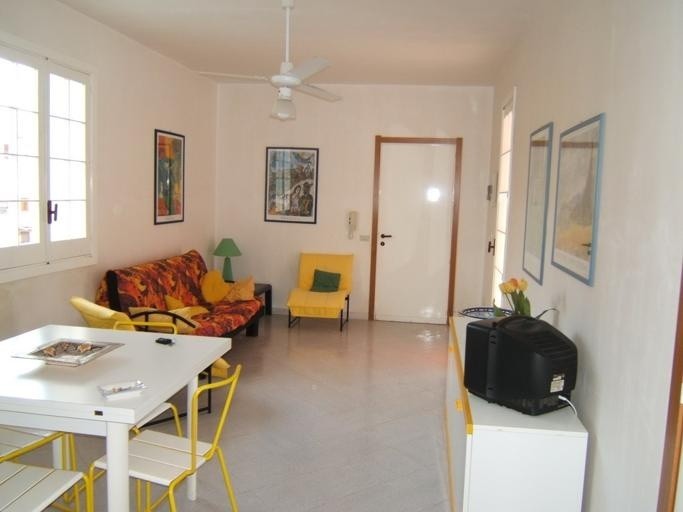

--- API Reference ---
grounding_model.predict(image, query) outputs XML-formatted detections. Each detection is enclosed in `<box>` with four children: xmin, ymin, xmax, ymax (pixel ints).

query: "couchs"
<box><xmin>96</xmin><ymin>249</ymin><xmax>263</xmax><ymax>382</ymax></box>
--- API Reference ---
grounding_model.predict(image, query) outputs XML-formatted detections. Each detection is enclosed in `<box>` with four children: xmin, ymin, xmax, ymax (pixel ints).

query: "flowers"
<box><xmin>457</xmin><ymin>277</ymin><xmax>560</xmax><ymax>319</ymax></box>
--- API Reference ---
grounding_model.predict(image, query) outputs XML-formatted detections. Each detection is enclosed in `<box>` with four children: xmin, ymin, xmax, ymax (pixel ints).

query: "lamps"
<box><xmin>213</xmin><ymin>238</ymin><xmax>242</xmax><ymax>281</ymax></box>
<box><xmin>269</xmin><ymin>87</ymin><xmax>296</xmax><ymax>121</ymax></box>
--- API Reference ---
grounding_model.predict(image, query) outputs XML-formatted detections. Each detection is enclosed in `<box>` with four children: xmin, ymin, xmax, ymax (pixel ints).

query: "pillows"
<box><xmin>129</xmin><ymin>268</ymin><xmax>255</xmax><ymax>335</ymax></box>
<box><xmin>310</xmin><ymin>269</ymin><xmax>341</xmax><ymax>292</ymax></box>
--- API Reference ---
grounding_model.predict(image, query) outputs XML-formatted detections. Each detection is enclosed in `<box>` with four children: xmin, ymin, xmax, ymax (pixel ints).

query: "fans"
<box><xmin>199</xmin><ymin>0</ymin><xmax>343</xmax><ymax>103</ymax></box>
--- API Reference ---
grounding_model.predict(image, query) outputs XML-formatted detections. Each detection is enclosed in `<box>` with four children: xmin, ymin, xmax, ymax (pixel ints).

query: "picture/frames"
<box><xmin>550</xmin><ymin>112</ymin><xmax>606</xmax><ymax>287</ymax></box>
<box><xmin>264</xmin><ymin>147</ymin><xmax>319</xmax><ymax>224</ymax></box>
<box><xmin>154</xmin><ymin>128</ymin><xmax>185</xmax><ymax>225</ymax></box>
<box><xmin>522</xmin><ymin>121</ymin><xmax>553</xmax><ymax>285</ymax></box>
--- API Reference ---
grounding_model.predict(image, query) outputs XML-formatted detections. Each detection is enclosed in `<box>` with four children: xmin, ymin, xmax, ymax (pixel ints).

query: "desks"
<box><xmin>0</xmin><ymin>324</ymin><xmax>231</xmax><ymax>512</ymax></box>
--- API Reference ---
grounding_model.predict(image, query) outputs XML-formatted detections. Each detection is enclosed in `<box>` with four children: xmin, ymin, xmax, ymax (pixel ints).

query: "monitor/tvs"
<box><xmin>464</xmin><ymin>315</ymin><xmax>578</xmax><ymax>415</ymax></box>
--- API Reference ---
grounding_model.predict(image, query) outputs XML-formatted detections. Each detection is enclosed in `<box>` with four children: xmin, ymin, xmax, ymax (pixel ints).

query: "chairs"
<box><xmin>286</xmin><ymin>252</ymin><xmax>353</xmax><ymax>332</ymax></box>
<box><xmin>0</xmin><ymin>423</ymin><xmax>80</xmax><ymax>512</ymax></box>
<box><xmin>61</xmin><ymin>321</ymin><xmax>183</xmax><ymax>504</ymax></box>
<box><xmin>70</xmin><ymin>295</ymin><xmax>231</xmax><ymax>428</ymax></box>
<box><xmin>0</xmin><ymin>461</ymin><xmax>90</xmax><ymax>512</ymax></box>
<box><xmin>89</xmin><ymin>364</ymin><xmax>242</xmax><ymax>512</ymax></box>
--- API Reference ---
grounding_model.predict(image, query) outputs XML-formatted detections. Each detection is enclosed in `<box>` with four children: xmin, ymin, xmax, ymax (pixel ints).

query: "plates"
<box><xmin>11</xmin><ymin>337</ymin><xmax>125</xmax><ymax>367</ymax></box>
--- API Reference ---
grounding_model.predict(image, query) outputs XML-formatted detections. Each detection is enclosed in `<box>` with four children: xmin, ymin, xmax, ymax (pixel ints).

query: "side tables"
<box><xmin>253</xmin><ymin>282</ymin><xmax>272</xmax><ymax>315</ymax></box>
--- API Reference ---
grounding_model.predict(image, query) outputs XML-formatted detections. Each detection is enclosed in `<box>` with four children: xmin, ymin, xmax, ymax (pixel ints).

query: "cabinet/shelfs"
<box><xmin>444</xmin><ymin>316</ymin><xmax>589</xmax><ymax>512</ymax></box>
